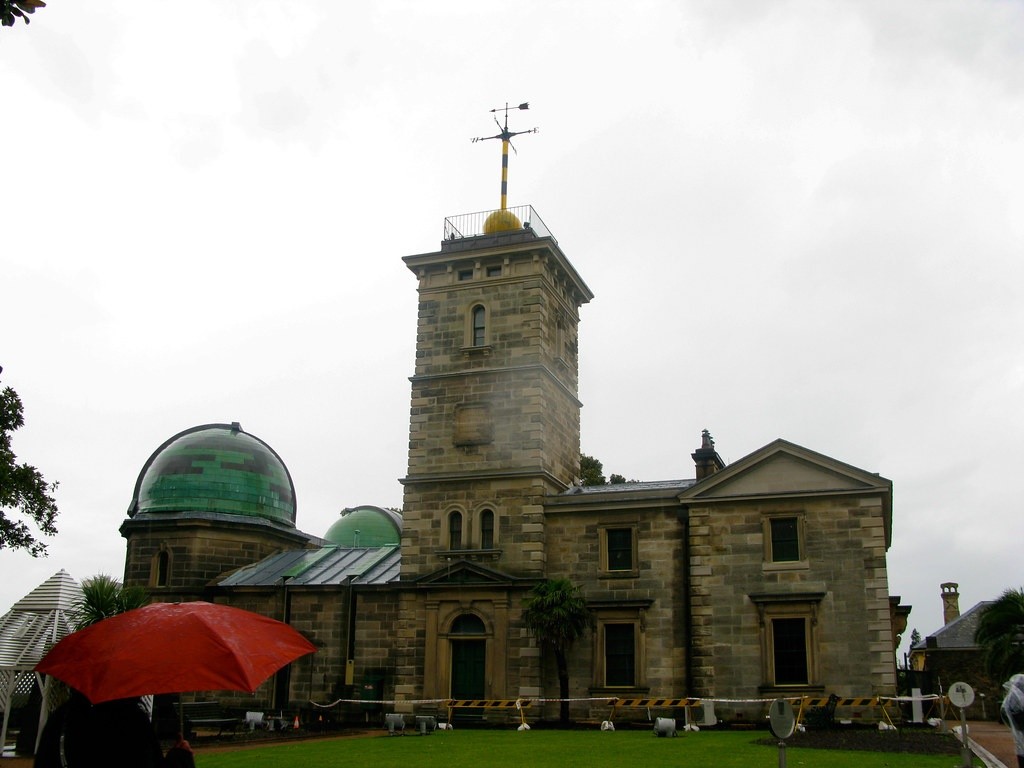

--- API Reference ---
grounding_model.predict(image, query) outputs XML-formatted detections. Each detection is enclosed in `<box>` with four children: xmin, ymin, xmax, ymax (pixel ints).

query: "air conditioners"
<box><xmin>685</xmin><ymin>701</ymin><xmax>717</xmax><ymax>726</ymax></box>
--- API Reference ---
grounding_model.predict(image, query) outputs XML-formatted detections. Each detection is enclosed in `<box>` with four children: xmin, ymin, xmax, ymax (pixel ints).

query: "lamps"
<box><xmin>381</xmin><ymin>713</ymin><xmax>406</xmax><ymax>736</ymax></box>
<box><xmin>414</xmin><ymin>716</ymin><xmax>437</xmax><ymax>736</ymax></box>
<box><xmin>652</xmin><ymin>717</ymin><xmax>680</xmax><ymax>738</ymax></box>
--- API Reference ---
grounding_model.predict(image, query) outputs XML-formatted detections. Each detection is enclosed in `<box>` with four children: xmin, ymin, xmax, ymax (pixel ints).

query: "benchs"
<box><xmin>174</xmin><ymin>701</ymin><xmax>241</xmax><ymax>741</ymax></box>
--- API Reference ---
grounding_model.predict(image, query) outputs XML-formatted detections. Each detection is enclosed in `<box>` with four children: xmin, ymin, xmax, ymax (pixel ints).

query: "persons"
<box><xmin>999</xmin><ymin>672</ymin><xmax>1024</xmax><ymax>768</ymax></box>
<box><xmin>33</xmin><ymin>696</ymin><xmax>197</xmax><ymax>768</ymax></box>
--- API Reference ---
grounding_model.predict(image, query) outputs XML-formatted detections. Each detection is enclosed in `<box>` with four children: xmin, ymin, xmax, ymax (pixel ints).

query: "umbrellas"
<box><xmin>30</xmin><ymin>601</ymin><xmax>319</xmax><ymax>709</ymax></box>
